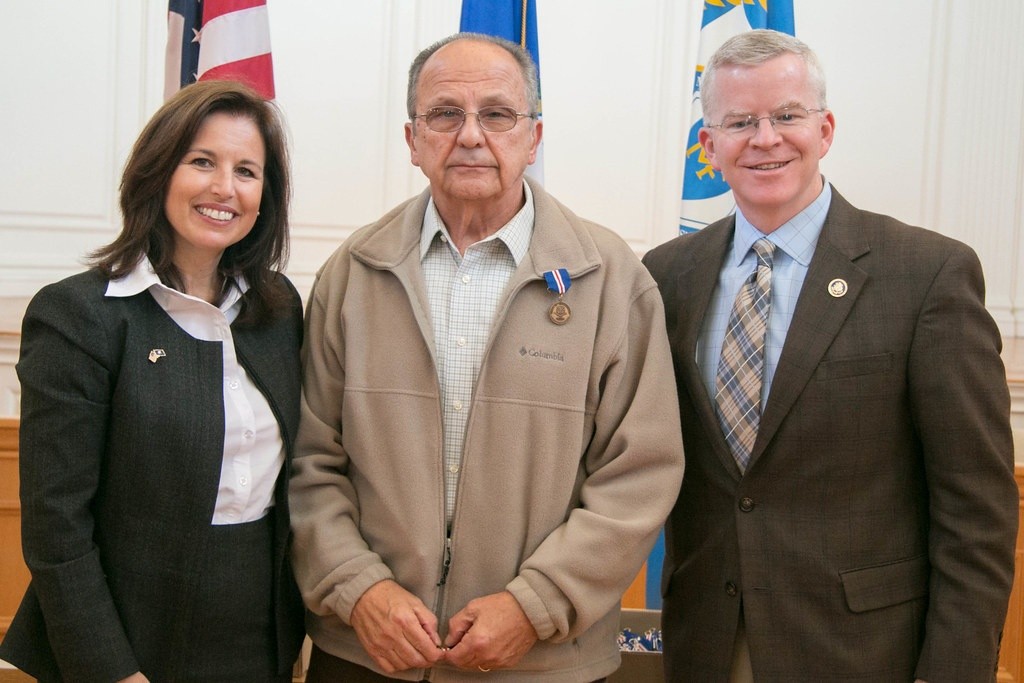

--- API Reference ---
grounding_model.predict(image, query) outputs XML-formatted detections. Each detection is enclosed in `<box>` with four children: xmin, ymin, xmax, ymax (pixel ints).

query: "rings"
<box><xmin>478</xmin><ymin>665</ymin><xmax>490</xmax><ymax>671</ymax></box>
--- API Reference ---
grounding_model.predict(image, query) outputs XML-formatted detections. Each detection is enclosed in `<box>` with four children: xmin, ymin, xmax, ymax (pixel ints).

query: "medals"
<box><xmin>548</xmin><ymin>295</ymin><xmax>572</xmax><ymax>324</ymax></box>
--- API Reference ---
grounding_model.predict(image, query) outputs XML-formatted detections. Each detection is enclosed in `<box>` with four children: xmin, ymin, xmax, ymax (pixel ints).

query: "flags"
<box><xmin>679</xmin><ymin>0</ymin><xmax>795</xmax><ymax>237</ymax></box>
<box><xmin>167</xmin><ymin>0</ymin><xmax>274</xmax><ymax>100</ymax></box>
<box><xmin>459</xmin><ymin>0</ymin><xmax>542</xmax><ymax>120</ymax></box>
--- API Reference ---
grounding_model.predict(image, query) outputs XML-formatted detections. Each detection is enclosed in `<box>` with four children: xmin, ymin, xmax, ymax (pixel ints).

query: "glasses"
<box><xmin>706</xmin><ymin>107</ymin><xmax>828</xmax><ymax>137</ymax></box>
<box><xmin>412</xmin><ymin>105</ymin><xmax>542</xmax><ymax>132</ymax></box>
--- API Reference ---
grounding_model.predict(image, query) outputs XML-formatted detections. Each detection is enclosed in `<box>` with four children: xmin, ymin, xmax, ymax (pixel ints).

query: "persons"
<box><xmin>640</xmin><ymin>27</ymin><xmax>1018</xmax><ymax>682</ymax></box>
<box><xmin>287</xmin><ymin>30</ymin><xmax>686</xmax><ymax>683</ymax></box>
<box><xmin>1</xmin><ymin>78</ymin><xmax>308</xmax><ymax>683</ymax></box>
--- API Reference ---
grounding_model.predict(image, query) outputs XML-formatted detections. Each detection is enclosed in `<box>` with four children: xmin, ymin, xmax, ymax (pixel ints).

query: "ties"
<box><xmin>714</xmin><ymin>237</ymin><xmax>776</xmax><ymax>479</ymax></box>
